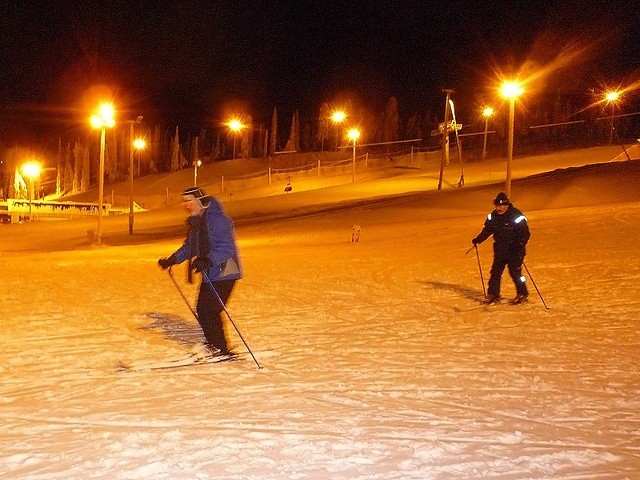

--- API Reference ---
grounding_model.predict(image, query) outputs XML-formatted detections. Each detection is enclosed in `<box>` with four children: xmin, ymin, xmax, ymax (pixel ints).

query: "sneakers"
<box><xmin>485</xmin><ymin>295</ymin><xmax>500</xmax><ymax>303</ymax></box>
<box><xmin>514</xmin><ymin>294</ymin><xmax>528</xmax><ymax>303</ymax></box>
<box><xmin>196</xmin><ymin>340</ymin><xmax>220</xmax><ymax>360</ymax></box>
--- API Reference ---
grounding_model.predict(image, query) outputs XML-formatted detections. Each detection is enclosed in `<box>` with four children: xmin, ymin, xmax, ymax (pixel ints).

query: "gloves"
<box><xmin>159</xmin><ymin>254</ymin><xmax>177</xmax><ymax>269</ymax></box>
<box><xmin>191</xmin><ymin>257</ymin><xmax>212</xmax><ymax>273</ymax></box>
<box><xmin>472</xmin><ymin>238</ymin><xmax>480</xmax><ymax>244</ymax></box>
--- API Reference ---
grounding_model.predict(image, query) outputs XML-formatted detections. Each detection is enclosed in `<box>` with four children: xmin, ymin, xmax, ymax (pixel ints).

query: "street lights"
<box><xmin>330</xmin><ymin>109</ymin><xmax>349</xmax><ymax>151</ymax></box>
<box><xmin>605</xmin><ymin>88</ymin><xmax>619</xmax><ymax>146</ymax></box>
<box><xmin>345</xmin><ymin>129</ymin><xmax>361</xmax><ymax>183</ymax></box>
<box><xmin>22</xmin><ymin>164</ymin><xmax>39</xmax><ymax>222</ymax></box>
<box><xmin>223</xmin><ymin>116</ymin><xmax>248</xmax><ymax>159</ymax></box>
<box><xmin>134</xmin><ymin>137</ymin><xmax>147</xmax><ymax>178</ymax></box>
<box><xmin>88</xmin><ymin>98</ymin><xmax>116</xmax><ymax>248</ymax></box>
<box><xmin>481</xmin><ymin>105</ymin><xmax>493</xmax><ymax>159</ymax></box>
<box><xmin>124</xmin><ymin>113</ymin><xmax>143</xmax><ymax>235</ymax></box>
<box><xmin>193</xmin><ymin>160</ymin><xmax>202</xmax><ymax>188</ymax></box>
<box><xmin>496</xmin><ymin>76</ymin><xmax>525</xmax><ymax>201</ymax></box>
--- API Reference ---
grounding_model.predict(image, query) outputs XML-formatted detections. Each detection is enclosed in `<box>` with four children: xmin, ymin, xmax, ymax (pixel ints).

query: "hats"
<box><xmin>495</xmin><ymin>192</ymin><xmax>509</xmax><ymax>205</ymax></box>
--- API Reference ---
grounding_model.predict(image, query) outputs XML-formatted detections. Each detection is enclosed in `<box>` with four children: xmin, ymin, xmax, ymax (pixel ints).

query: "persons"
<box><xmin>472</xmin><ymin>192</ymin><xmax>531</xmax><ymax>306</ymax></box>
<box><xmin>159</xmin><ymin>186</ymin><xmax>242</xmax><ymax>358</ymax></box>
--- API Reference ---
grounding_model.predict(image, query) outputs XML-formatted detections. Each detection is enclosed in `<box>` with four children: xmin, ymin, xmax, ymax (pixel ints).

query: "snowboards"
<box><xmin>455</xmin><ymin>299</ymin><xmax>536</xmax><ymax>312</ymax></box>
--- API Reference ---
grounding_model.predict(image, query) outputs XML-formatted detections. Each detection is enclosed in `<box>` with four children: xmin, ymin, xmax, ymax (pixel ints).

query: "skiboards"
<box><xmin>151</xmin><ymin>348</ymin><xmax>249</xmax><ymax>366</ymax></box>
